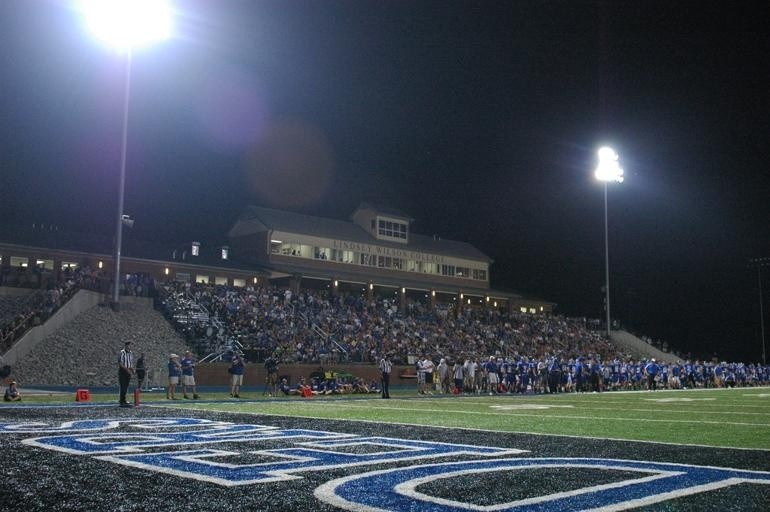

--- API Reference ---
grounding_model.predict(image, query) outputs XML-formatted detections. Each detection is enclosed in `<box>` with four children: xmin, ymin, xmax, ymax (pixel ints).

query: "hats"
<box><xmin>168</xmin><ymin>354</ymin><xmax>180</xmax><ymax>359</ymax></box>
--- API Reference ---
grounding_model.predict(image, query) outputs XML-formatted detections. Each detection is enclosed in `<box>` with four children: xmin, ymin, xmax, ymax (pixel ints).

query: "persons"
<box><xmin>0</xmin><ymin>260</ymin><xmax>113</xmax><ymax>356</ymax></box>
<box><xmin>292</xmin><ymin>250</ymin><xmax>399</xmax><ymax>269</ymax></box>
<box><xmin>115</xmin><ymin>340</ymin><xmax>135</xmax><ymax>409</ymax></box>
<box><xmin>136</xmin><ymin>352</ymin><xmax>149</xmax><ymax>389</ymax></box>
<box><xmin>378</xmin><ymin>354</ymin><xmax>393</xmax><ymax>399</ymax></box>
<box><xmin>165</xmin><ymin>350</ymin><xmax>201</xmax><ymax>400</ymax></box>
<box><xmin>119</xmin><ymin>273</ymin><xmax>693</xmax><ymax>366</ymax></box>
<box><xmin>414</xmin><ymin>353</ymin><xmax>769</xmax><ymax>396</ymax></box>
<box><xmin>262</xmin><ymin>353</ymin><xmax>382</xmax><ymax>397</ymax></box>
<box><xmin>3</xmin><ymin>381</ymin><xmax>22</xmax><ymax>402</ymax></box>
<box><xmin>228</xmin><ymin>350</ymin><xmax>246</xmax><ymax>398</ymax></box>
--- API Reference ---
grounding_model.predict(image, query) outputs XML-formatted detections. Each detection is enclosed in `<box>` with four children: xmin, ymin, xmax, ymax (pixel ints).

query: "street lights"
<box><xmin>594</xmin><ymin>146</ymin><xmax>623</xmax><ymax>337</ymax></box>
<box><xmin>94</xmin><ymin>2</ymin><xmax>158</xmax><ymax>302</ymax></box>
